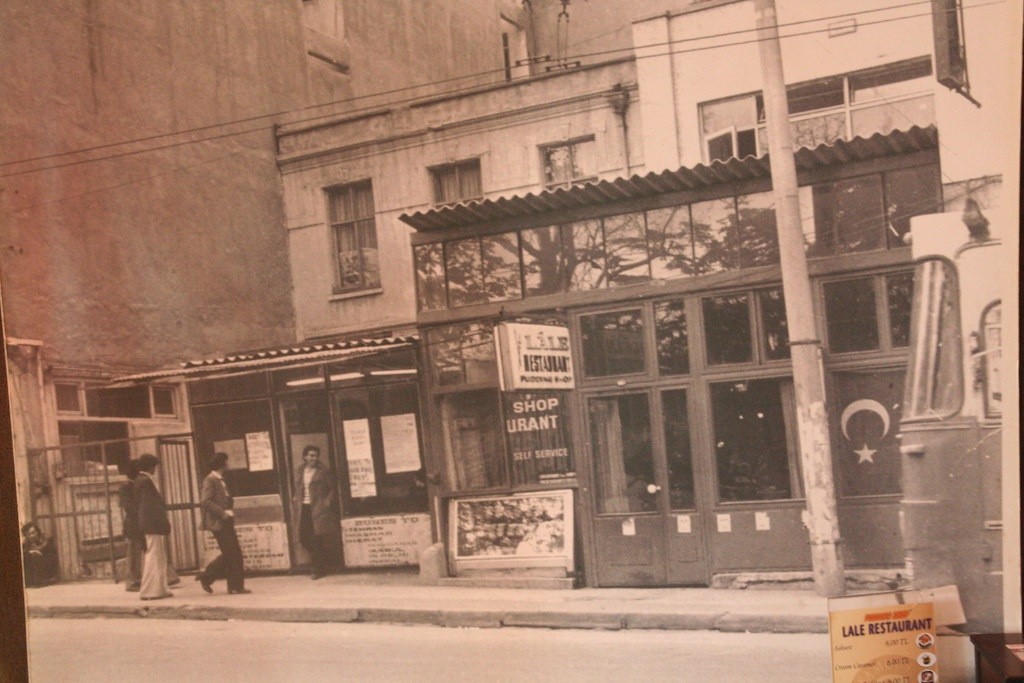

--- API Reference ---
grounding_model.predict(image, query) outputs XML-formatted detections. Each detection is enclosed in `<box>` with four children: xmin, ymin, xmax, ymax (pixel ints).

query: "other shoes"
<box><xmin>311</xmin><ymin>573</ymin><xmax>318</xmax><ymax>580</ymax></box>
<box><xmin>227</xmin><ymin>585</ymin><xmax>251</xmax><ymax>594</ymax></box>
<box><xmin>195</xmin><ymin>574</ymin><xmax>213</xmax><ymax>593</ymax></box>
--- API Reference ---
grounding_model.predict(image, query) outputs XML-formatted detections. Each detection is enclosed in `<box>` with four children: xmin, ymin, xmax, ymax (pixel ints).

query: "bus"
<box><xmin>898</xmin><ymin>207</ymin><xmax>1023</xmax><ymax>642</ymax></box>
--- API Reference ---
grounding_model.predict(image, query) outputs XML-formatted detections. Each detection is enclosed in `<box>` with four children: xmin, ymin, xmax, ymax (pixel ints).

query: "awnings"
<box><xmin>112</xmin><ymin>342</ymin><xmax>418</xmax><ymax>382</ymax></box>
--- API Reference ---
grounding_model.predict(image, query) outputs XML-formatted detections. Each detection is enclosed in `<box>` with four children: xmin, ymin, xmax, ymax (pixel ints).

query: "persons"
<box><xmin>21</xmin><ymin>522</ymin><xmax>59</xmax><ymax>587</ymax></box>
<box><xmin>118</xmin><ymin>453</ymin><xmax>181</xmax><ymax>601</ymax></box>
<box><xmin>195</xmin><ymin>452</ymin><xmax>252</xmax><ymax>595</ymax></box>
<box><xmin>292</xmin><ymin>445</ymin><xmax>337</xmax><ymax>580</ymax></box>
